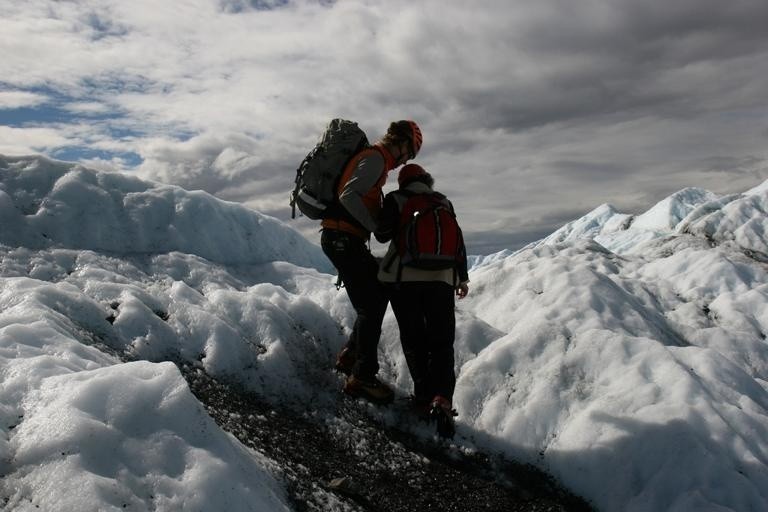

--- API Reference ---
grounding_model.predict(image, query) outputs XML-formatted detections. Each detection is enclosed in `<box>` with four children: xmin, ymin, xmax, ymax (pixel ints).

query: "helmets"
<box><xmin>405</xmin><ymin>120</ymin><xmax>423</xmax><ymax>158</ymax></box>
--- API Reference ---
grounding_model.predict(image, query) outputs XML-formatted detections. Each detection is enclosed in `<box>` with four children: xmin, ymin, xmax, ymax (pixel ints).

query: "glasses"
<box><xmin>407</xmin><ymin>142</ymin><xmax>415</xmax><ymax>159</ymax></box>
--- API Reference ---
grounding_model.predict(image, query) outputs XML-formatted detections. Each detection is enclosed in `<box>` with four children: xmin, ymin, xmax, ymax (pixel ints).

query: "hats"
<box><xmin>398</xmin><ymin>163</ymin><xmax>425</xmax><ymax>182</ymax></box>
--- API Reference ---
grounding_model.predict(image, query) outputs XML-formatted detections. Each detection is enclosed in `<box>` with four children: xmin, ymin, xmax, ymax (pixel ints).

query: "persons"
<box><xmin>321</xmin><ymin>119</ymin><xmax>422</xmax><ymax>405</ymax></box>
<box><xmin>374</xmin><ymin>163</ymin><xmax>470</xmax><ymax>439</ymax></box>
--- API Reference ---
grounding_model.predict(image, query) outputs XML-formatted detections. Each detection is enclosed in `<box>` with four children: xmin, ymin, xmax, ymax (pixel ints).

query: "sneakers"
<box><xmin>335</xmin><ymin>344</ymin><xmax>395</xmax><ymax>405</ymax></box>
<box><xmin>414</xmin><ymin>394</ymin><xmax>458</xmax><ymax>439</ymax></box>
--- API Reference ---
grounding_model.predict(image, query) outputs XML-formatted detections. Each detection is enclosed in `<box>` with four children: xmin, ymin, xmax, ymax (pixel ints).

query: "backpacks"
<box><xmin>290</xmin><ymin>119</ymin><xmax>386</xmax><ymax>220</ymax></box>
<box><xmin>393</xmin><ymin>188</ymin><xmax>461</xmax><ymax>271</ymax></box>
